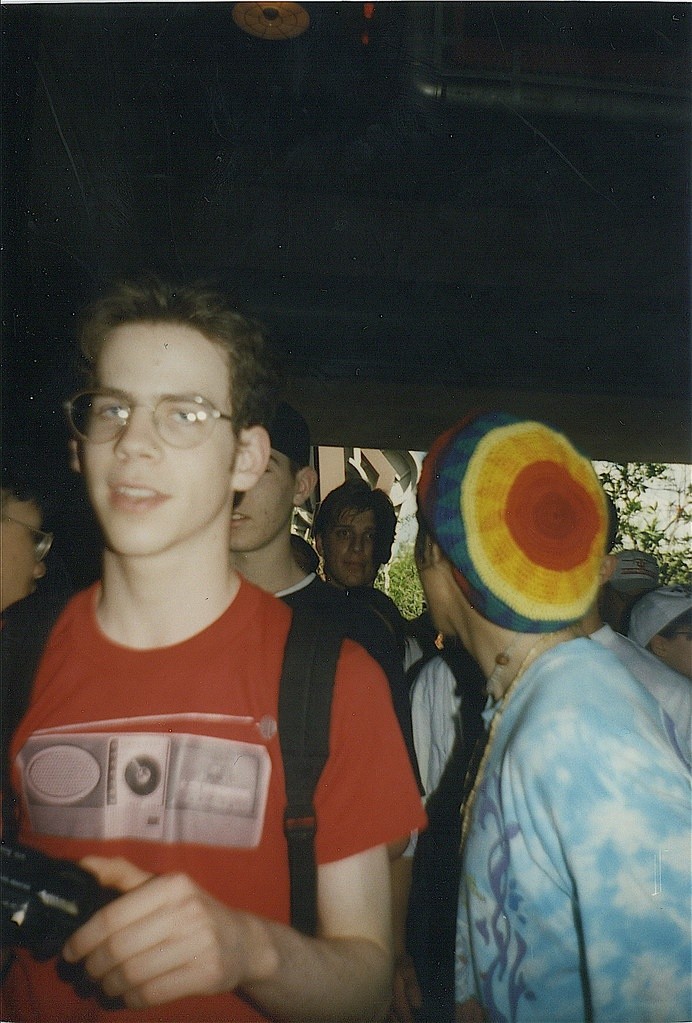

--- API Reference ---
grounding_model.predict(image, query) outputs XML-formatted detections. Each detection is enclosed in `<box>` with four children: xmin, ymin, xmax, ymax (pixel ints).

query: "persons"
<box><xmin>414</xmin><ymin>408</ymin><xmax>692</xmax><ymax>1022</ymax></box>
<box><xmin>0</xmin><ymin>273</ymin><xmax>427</xmax><ymax>1023</ymax></box>
<box><xmin>392</xmin><ymin>494</ymin><xmax>616</xmax><ymax>1022</ymax></box>
<box><xmin>0</xmin><ymin>468</ymin><xmax>53</xmax><ymax>627</ymax></box>
<box><xmin>605</xmin><ymin>549</ymin><xmax>692</xmax><ymax>683</ymax></box>
<box><xmin>313</xmin><ymin>481</ymin><xmax>397</xmax><ymax>590</ymax></box>
<box><xmin>228</xmin><ymin>403</ymin><xmax>425</xmax><ymax>855</ymax></box>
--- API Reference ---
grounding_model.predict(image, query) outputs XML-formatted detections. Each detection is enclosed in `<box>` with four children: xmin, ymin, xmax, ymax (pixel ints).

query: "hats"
<box><xmin>609</xmin><ymin>550</ymin><xmax>660</xmax><ymax>594</ymax></box>
<box><xmin>417</xmin><ymin>410</ymin><xmax>607</xmax><ymax>634</ymax></box>
<box><xmin>267</xmin><ymin>403</ymin><xmax>311</xmax><ymax>468</ymax></box>
<box><xmin>628</xmin><ymin>584</ymin><xmax>692</xmax><ymax>648</ymax></box>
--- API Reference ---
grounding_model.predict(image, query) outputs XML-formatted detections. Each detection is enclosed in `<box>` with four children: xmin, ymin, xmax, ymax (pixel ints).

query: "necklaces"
<box><xmin>458</xmin><ymin>630</ymin><xmax>559</xmax><ymax>853</ymax></box>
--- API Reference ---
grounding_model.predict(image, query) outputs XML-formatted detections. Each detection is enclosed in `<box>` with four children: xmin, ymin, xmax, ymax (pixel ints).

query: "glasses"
<box><xmin>62</xmin><ymin>387</ymin><xmax>236</xmax><ymax>448</ymax></box>
<box><xmin>0</xmin><ymin>514</ymin><xmax>54</xmax><ymax>562</ymax></box>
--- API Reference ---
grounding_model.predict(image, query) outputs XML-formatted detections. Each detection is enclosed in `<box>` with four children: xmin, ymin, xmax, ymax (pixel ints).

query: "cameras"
<box><xmin>1</xmin><ymin>837</ymin><xmax>114</xmax><ymax>958</ymax></box>
